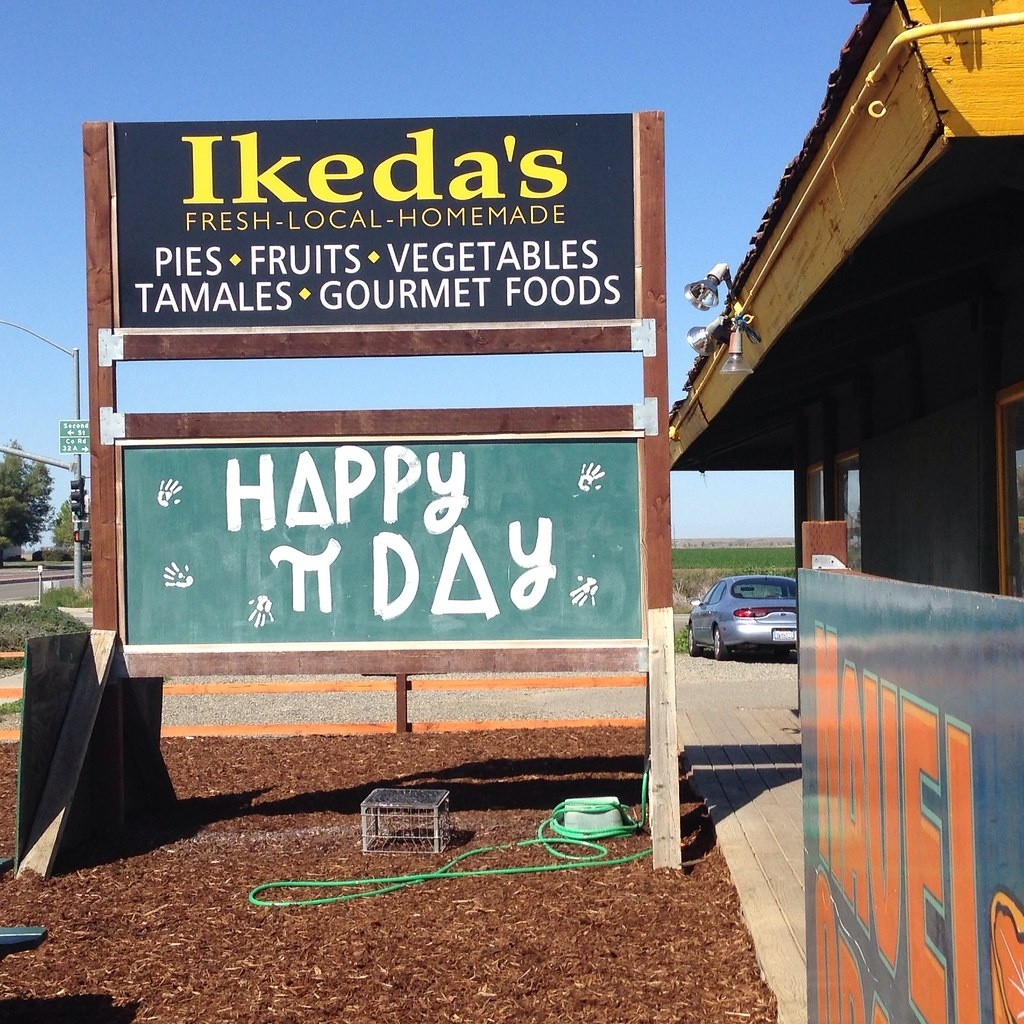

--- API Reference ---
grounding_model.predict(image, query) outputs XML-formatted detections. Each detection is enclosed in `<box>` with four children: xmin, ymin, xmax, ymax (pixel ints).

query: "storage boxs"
<box><xmin>562</xmin><ymin>794</ymin><xmax>625</xmax><ymax>835</ymax></box>
<box><xmin>361</xmin><ymin>785</ymin><xmax>451</xmax><ymax>855</ymax></box>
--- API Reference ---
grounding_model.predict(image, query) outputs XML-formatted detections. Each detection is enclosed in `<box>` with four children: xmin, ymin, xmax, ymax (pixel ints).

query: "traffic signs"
<box><xmin>59</xmin><ymin>420</ymin><xmax>89</xmax><ymax>437</ymax></box>
<box><xmin>59</xmin><ymin>437</ymin><xmax>90</xmax><ymax>454</ymax></box>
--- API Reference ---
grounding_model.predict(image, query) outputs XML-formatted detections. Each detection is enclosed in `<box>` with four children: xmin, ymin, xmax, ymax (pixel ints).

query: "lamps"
<box><xmin>678</xmin><ymin>254</ymin><xmax>736</xmax><ymax>314</ymax></box>
<box><xmin>717</xmin><ymin>323</ymin><xmax>753</xmax><ymax>379</ymax></box>
<box><xmin>685</xmin><ymin>318</ymin><xmax>731</xmax><ymax>360</ymax></box>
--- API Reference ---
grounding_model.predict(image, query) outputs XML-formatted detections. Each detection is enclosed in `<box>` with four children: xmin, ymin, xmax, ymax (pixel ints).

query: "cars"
<box><xmin>688</xmin><ymin>575</ymin><xmax>798</xmax><ymax>661</ymax></box>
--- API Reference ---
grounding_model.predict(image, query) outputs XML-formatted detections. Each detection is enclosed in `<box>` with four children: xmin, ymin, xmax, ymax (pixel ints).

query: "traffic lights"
<box><xmin>70</xmin><ymin>475</ymin><xmax>86</xmax><ymax>520</ymax></box>
